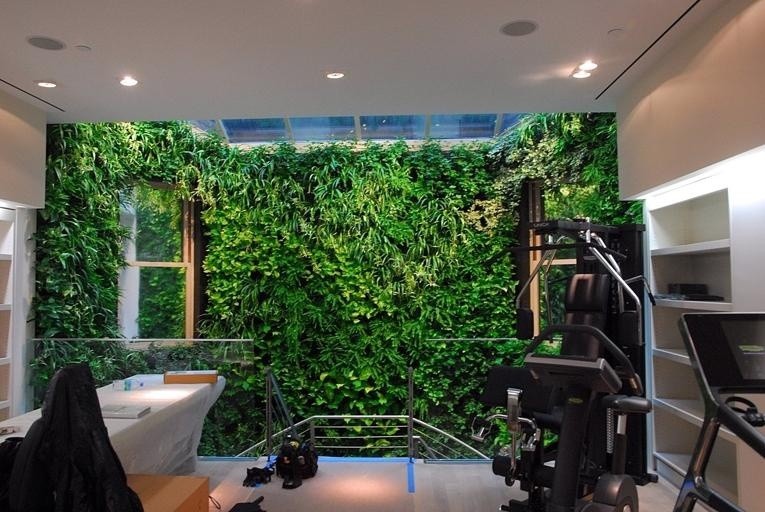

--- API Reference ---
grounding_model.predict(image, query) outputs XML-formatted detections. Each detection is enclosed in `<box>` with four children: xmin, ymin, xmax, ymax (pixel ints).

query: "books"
<box><xmin>656</xmin><ymin>281</ymin><xmax>727</xmax><ymax>301</ymax></box>
<box><xmin>101</xmin><ymin>404</ymin><xmax>152</xmax><ymax>418</ymax></box>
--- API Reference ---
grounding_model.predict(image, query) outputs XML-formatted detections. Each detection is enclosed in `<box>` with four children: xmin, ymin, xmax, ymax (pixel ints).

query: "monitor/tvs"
<box><xmin>523</xmin><ymin>351</ymin><xmax>623</xmax><ymax>394</ymax></box>
<box><xmin>677</xmin><ymin>311</ymin><xmax>765</xmax><ymax>392</ymax></box>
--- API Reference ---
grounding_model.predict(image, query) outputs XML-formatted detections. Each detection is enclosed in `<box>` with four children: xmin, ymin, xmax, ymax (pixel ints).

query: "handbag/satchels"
<box><xmin>276</xmin><ymin>443</ymin><xmax>318</xmax><ymax>479</ymax></box>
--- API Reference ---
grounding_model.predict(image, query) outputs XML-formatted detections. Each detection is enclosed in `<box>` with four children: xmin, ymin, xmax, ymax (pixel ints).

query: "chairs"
<box><xmin>0</xmin><ymin>362</ymin><xmax>143</xmax><ymax>512</ymax></box>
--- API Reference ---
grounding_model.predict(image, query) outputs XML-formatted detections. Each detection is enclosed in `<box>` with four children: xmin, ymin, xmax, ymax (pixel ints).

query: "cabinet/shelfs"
<box><xmin>0</xmin><ymin>208</ymin><xmax>47</xmax><ymax>419</ymax></box>
<box><xmin>642</xmin><ymin>175</ymin><xmax>740</xmax><ymax>512</ymax></box>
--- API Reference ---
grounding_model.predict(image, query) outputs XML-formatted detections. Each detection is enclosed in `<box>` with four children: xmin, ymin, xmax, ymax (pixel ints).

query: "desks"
<box><xmin>0</xmin><ymin>374</ymin><xmax>226</xmax><ymax>477</ymax></box>
<box><xmin>123</xmin><ymin>473</ymin><xmax>209</xmax><ymax>512</ymax></box>
